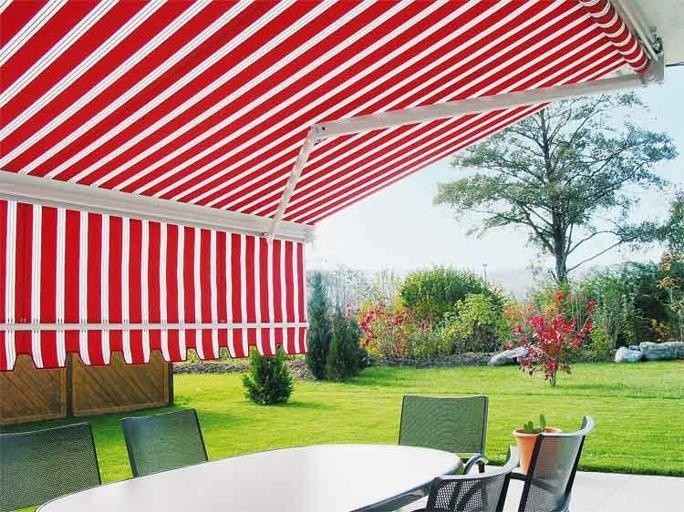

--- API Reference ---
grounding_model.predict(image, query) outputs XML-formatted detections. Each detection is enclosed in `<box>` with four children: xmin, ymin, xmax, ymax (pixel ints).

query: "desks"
<box><xmin>36</xmin><ymin>444</ymin><xmax>464</xmax><ymax>512</ymax></box>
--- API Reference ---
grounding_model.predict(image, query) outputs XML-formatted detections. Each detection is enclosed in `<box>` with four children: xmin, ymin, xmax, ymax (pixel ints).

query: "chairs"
<box><xmin>399</xmin><ymin>396</ymin><xmax>594</xmax><ymax>512</ymax></box>
<box><xmin>120</xmin><ymin>409</ymin><xmax>208</xmax><ymax>477</ymax></box>
<box><xmin>0</xmin><ymin>422</ymin><xmax>101</xmax><ymax>512</ymax></box>
